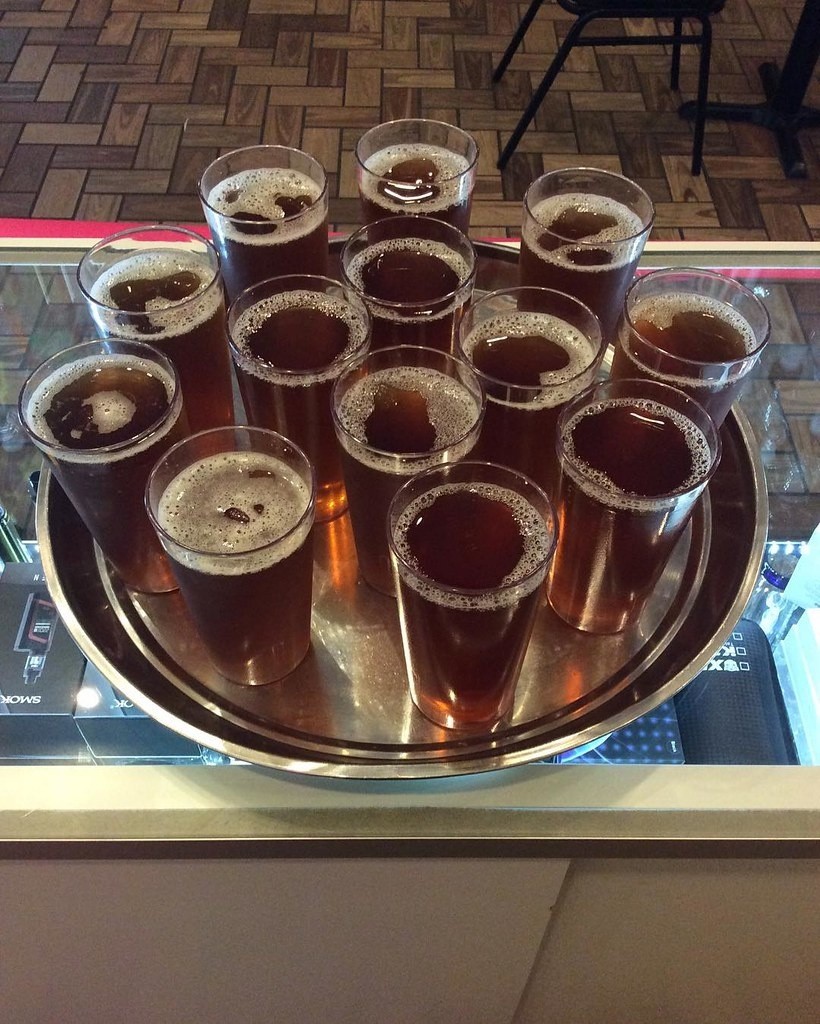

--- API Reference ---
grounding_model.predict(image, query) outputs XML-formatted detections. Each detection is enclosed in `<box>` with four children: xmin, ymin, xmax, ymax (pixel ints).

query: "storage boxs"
<box><xmin>554</xmin><ymin>697</ymin><xmax>685</xmax><ymax>765</ymax></box>
<box><xmin>0</xmin><ymin>559</ymin><xmax>203</xmax><ymax>758</ymax></box>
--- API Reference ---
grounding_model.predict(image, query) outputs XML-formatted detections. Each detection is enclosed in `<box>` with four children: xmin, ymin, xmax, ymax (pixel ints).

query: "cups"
<box><xmin>198</xmin><ymin>144</ymin><xmax>329</xmax><ymax>313</ymax></box>
<box><xmin>606</xmin><ymin>267</ymin><xmax>772</xmax><ymax>433</ymax></box>
<box><xmin>339</xmin><ymin>214</ymin><xmax>479</xmax><ymax>379</ymax></box>
<box><xmin>223</xmin><ymin>272</ymin><xmax>374</xmax><ymax>523</ymax></box>
<box><xmin>452</xmin><ymin>285</ymin><xmax>607</xmax><ymax>504</ymax></box>
<box><xmin>330</xmin><ymin>344</ymin><xmax>487</xmax><ymax>601</ymax></box>
<box><xmin>546</xmin><ymin>377</ymin><xmax>722</xmax><ymax>633</ymax></box>
<box><xmin>383</xmin><ymin>459</ymin><xmax>561</xmax><ymax>734</ymax></box>
<box><xmin>515</xmin><ymin>165</ymin><xmax>656</xmax><ymax>346</ymax></box>
<box><xmin>354</xmin><ymin>117</ymin><xmax>482</xmax><ymax>249</ymax></box>
<box><xmin>17</xmin><ymin>336</ymin><xmax>194</xmax><ymax>594</ymax></box>
<box><xmin>143</xmin><ymin>426</ymin><xmax>315</xmax><ymax>685</ymax></box>
<box><xmin>74</xmin><ymin>223</ymin><xmax>236</xmax><ymax>461</ymax></box>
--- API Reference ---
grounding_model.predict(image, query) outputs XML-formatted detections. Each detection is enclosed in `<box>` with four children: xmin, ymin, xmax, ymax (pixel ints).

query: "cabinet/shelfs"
<box><xmin>0</xmin><ymin>215</ymin><xmax>819</xmax><ymax>1024</ymax></box>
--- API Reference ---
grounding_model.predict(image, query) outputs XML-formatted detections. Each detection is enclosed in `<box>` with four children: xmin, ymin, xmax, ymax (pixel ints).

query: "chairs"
<box><xmin>491</xmin><ymin>0</ymin><xmax>726</xmax><ymax>176</ymax></box>
